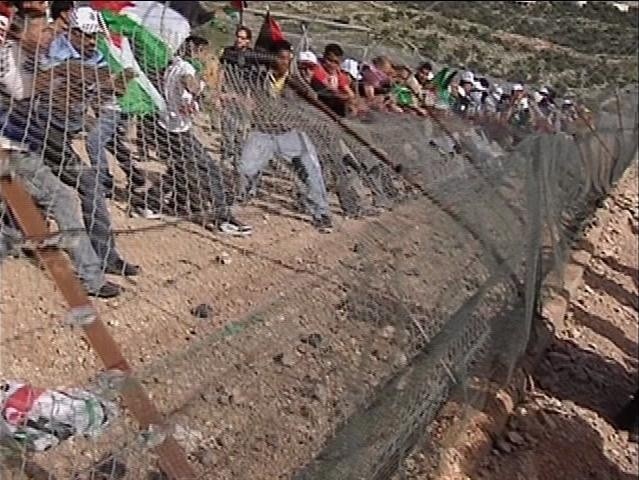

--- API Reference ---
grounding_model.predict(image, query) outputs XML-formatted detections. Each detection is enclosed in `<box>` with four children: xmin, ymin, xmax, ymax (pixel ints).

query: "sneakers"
<box><xmin>313</xmin><ymin>215</ymin><xmax>335</xmax><ymax>230</ymax></box>
<box><xmin>104</xmin><ymin>260</ymin><xmax>141</xmax><ymax>276</ymax></box>
<box><xmin>207</xmin><ymin>214</ymin><xmax>251</xmax><ymax>234</ymax></box>
<box><xmin>88</xmin><ymin>282</ymin><xmax>125</xmax><ymax>297</ymax></box>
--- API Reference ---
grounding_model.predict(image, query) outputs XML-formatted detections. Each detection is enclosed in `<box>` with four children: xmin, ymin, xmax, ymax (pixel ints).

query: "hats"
<box><xmin>68</xmin><ymin>6</ymin><xmax>106</xmax><ymax>34</ymax></box>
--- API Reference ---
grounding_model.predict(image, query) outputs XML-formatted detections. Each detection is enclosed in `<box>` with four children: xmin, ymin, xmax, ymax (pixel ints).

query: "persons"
<box><xmin>364</xmin><ymin>52</ymin><xmax>595</xmax><ymax>144</ymax></box>
